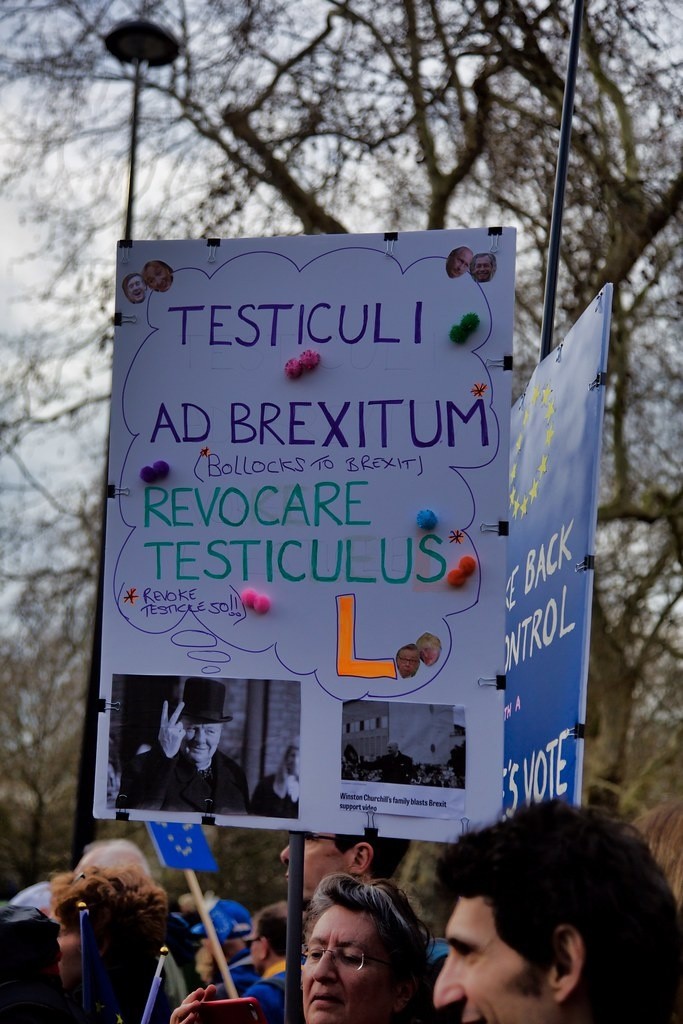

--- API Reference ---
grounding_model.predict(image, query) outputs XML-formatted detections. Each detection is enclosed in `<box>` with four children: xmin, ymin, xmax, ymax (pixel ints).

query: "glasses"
<box><xmin>71</xmin><ymin>872</ymin><xmax>86</xmax><ymax>885</ymax></box>
<box><xmin>301</xmin><ymin>943</ymin><xmax>395</xmax><ymax>970</ymax></box>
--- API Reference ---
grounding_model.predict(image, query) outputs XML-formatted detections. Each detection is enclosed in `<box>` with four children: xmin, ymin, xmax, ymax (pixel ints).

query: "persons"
<box><xmin>0</xmin><ymin>801</ymin><xmax>683</xmax><ymax>1024</ymax></box>
<box><xmin>107</xmin><ymin>677</ymin><xmax>467</xmax><ymax>818</ymax></box>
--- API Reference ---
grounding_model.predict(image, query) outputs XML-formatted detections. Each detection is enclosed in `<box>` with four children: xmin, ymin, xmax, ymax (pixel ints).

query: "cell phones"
<box><xmin>198</xmin><ymin>997</ymin><xmax>270</xmax><ymax>1024</ymax></box>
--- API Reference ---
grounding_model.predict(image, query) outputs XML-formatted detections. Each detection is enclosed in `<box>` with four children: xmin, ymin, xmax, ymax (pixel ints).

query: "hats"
<box><xmin>190</xmin><ymin>900</ymin><xmax>252</xmax><ymax>940</ymax></box>
<box><xmin>165</xmin><ymin>678</ymin><xmax>234</xmax><ymax>723</ymax></box>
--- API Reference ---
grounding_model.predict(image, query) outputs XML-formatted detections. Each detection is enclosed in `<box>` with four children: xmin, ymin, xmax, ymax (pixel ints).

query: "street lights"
<box><xmin>68</xmin><ymin>19</ymin><xmax>180</xmax><ymax>878</ymax></box>
